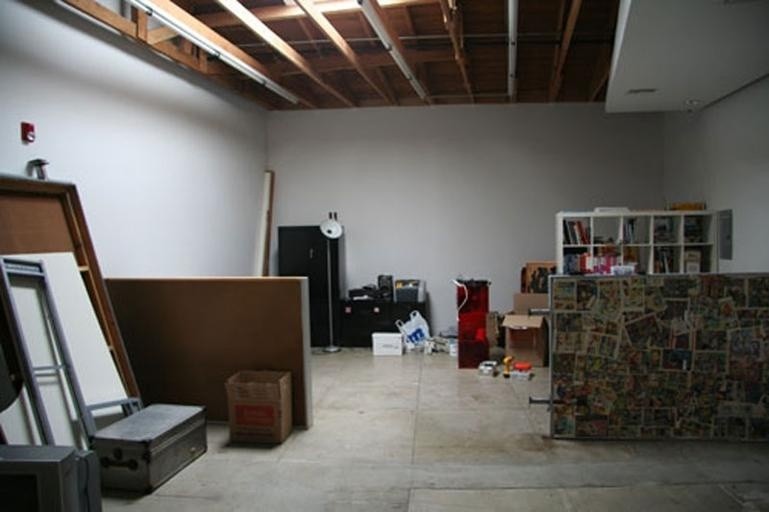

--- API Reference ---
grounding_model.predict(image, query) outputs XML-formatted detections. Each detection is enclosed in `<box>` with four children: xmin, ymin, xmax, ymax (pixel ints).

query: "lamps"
<box><xmin>318</xmin><ymin>220</ymin><xmax>345</xmax><ymax>355</ymax></box>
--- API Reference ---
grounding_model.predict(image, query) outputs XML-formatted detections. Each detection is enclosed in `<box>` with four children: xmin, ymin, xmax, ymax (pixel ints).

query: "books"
<box><xmin>563</xmin><ymin>218</ymin><xmax>706</xmax><ymax>276</ymax></box>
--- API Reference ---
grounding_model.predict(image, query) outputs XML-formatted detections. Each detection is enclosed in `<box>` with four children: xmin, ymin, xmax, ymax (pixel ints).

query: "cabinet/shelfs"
<box><xmin>277</xmin><ymin>224</ymin><xmax>346</xmax><ymax>349</ymax></box>
<box><xmin>336</xmin><ymin>297</ymin><xmax>428</xmax><ymax>348</ymax></box>
<box><xmin>556</xmin><ymin>209</ymin><xmax>721</xmax><ymax>276</ymax></box>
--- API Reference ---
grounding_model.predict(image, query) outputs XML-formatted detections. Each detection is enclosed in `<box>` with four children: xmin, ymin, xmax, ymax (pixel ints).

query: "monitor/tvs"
<box><xmin>0</xmin><ymin>443</ymin><xmax>103</xmax><ymax>512</ymax></box>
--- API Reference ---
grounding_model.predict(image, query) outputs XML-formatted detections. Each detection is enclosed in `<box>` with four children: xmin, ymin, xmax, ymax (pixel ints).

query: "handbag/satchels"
<box><xmin>394</xmin><ymin>309</ymin><xmax>433</xmax><ymax>355</ymax></box>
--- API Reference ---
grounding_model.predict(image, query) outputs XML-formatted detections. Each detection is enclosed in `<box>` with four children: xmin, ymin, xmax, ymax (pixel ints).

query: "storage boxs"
<box><xmin>504</xmin><ymin>291</ymin><xmax>549</xmax><ymax>367</ymax></box>
<box><xmin>88</xmin><ymin>402</ymin><xmax>207</xmax><ymax>495</ymax></box>
<box><xmin>226</xmin><ymin>370</ymin><xmax>296</xmax><ymax>446</ymax></box>
